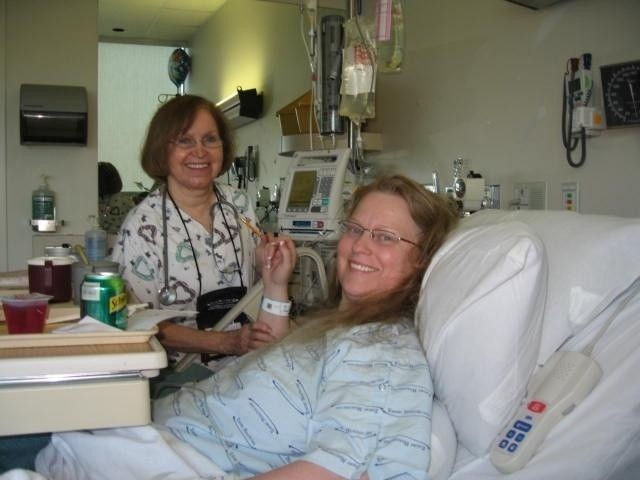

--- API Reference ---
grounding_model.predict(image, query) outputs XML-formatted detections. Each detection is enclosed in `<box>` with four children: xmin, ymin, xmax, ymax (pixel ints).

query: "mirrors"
<box><xmin>97</xmin><ymin>40</ymin><xmax>189</xmax><ymax>256</ymax></box>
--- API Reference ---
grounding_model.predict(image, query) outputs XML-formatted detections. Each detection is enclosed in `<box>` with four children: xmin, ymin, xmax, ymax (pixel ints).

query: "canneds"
<box><xmin>80</xmin><ymin>272</ymin><xmax>130</xmax><ymax>331</ymax></box>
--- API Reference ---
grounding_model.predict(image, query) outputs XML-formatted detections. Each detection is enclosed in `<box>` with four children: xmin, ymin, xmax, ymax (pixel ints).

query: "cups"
<box><xmin>73</xmin><ymin>261</ymin><xmax>121</xmax><ymax>305</ymax></box>
<box><xmin>26</xmin><ymin>256</ymin><xmax>74</xmax><ymax>304</ymax></box>
<box><xmin>43</xmin><ymin>246</ymin><xmax>72</xmax><ymax>256</ymax></box>
<box><xmin>0</xmin><ymin>294</ymin><xmax>51</xmax><ymax>335</ymax></box>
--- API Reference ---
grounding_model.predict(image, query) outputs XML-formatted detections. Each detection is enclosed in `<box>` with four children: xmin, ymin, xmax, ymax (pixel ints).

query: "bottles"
<box><xmin>445</xmin><ymin>179</ymin><xmax>456</xmax><ymax>198</ymax></box>
<box><xmin>84</xmin><ymin>223</ymin><xmax>108</xmax><ymax>264</ymax></box>
<box><xmin>278</xmin><ymin>176</ymin><xmax>285</xmax><ymax>198</ymax></box>
<box><xmin>455</xmin><ymin>152</ymin><xmax>464</xmax><ymax>177</ymax></box>
<box><xmin>452</xmin><ymin>158</ymin><xmax>458</xmax><ymax>178</ymax></box>
<box><xmin>491</xmin><ymin>183</ymin><xmax>500</xmax><ymax>210</ymax></box>
<box><xmin>271</xmin><ymin>184</ymin><xmax>280</xmax><ymax>202</ymax></box>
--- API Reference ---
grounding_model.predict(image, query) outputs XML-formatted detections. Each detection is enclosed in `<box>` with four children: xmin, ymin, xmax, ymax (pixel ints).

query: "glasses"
<box><xmin>169</xmin><ymin>135</ymin><xmax>224</xmax><ymax>148</ymax></box>
<box><xmin>341</xmin><ymin>220</ymin><xmax>419</xmax><ymax>246</ymax></box>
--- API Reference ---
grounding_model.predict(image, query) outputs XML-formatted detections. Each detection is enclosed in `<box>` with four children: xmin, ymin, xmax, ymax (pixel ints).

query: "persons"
<box><xmin>111</xmin><ymin>94</ymin><xmax>276</xmax><ymax>399</ymax></box>
<box><xmin>151</xmin><ymin>174</ymin><xmax>460</xmax><ymax>480</ymax></box>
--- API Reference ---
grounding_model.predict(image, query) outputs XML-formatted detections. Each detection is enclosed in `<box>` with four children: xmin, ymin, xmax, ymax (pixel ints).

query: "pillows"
<box><xmin>429</xmin><ymin>209</ymin><xmax>640</xmax><ymax>365</ymax></box>
<box><xmin>414</xmin><ymin>220</ymin><xmax>547</xmax><ymax>458</ymax></box>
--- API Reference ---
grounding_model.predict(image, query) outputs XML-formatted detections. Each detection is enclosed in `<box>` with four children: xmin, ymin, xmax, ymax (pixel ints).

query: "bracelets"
<box><xmin>261</xmin><ymin>298</ymin><xmax>292</xmax><ymax>317</ymax></box>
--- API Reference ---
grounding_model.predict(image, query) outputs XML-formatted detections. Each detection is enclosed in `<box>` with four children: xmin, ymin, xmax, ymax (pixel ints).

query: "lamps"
<box><xmin>215</xmin><ymin>86</ymin><xmax>263</xmax><ymax>130</ymax></box>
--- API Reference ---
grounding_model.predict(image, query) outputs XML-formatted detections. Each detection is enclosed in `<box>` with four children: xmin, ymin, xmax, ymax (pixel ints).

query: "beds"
<box><xmin>152</xmin><ymin>209</ymin><xmax>640</xmax><ymax>480</ymax></box>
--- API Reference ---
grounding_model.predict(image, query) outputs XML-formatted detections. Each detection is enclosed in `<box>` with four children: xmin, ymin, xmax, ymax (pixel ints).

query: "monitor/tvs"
<box><xmin>279</xmin><ymin>165</ymin><xmax>342</xmax><ymax>219</ymax></box>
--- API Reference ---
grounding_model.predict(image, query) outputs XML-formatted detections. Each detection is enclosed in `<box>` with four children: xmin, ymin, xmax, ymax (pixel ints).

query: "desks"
<box><xmin>0</xmin><ymin>335</ymin><xmax>167</xmax><ymax>437</ymax></box>
